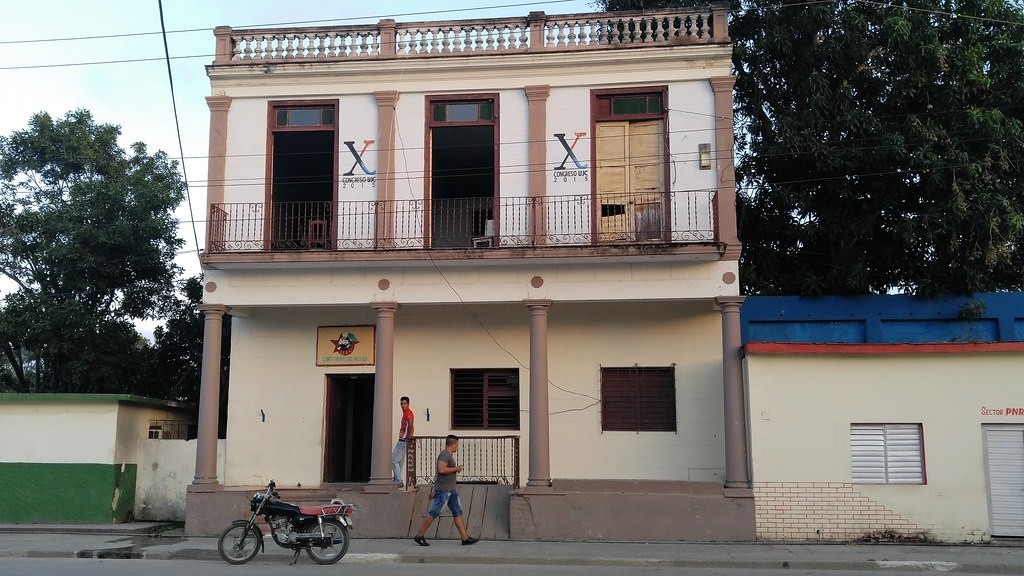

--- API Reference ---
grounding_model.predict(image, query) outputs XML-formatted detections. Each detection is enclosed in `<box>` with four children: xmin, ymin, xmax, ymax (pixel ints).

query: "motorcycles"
<box><xmin>217</xmin><ymin>480</ymin><xmax>356</xmax><ymax>565</ymax></box>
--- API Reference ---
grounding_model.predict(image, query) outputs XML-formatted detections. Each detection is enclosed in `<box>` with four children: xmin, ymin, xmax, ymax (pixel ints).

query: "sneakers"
<box><xmin>414</xmin><ymin>535</ymin><xmax>429</xmax><ymax>546</ymax></box>
<box><xmin>462</xmin><ymin>537</ymin><xmax>479</xmax><ymax>545</ymax></box>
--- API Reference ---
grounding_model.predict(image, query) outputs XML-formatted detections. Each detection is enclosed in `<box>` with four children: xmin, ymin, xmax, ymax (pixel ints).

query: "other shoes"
<box><xmin>397</xmin><ymin>482</ymin><xmax>404</xmax><ymax>488</ymax></box>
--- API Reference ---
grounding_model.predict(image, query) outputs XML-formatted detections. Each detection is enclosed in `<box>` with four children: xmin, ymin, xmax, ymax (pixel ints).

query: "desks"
<box><xmin>472</xmin><ymin>236</ymin><xmax>493</xmax><ymax>249</ymax></box>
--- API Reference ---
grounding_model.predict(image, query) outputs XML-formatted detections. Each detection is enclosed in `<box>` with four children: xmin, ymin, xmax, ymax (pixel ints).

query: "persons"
<box><xmin>391</xmin><ymin>397</ymin><xmax>414</xmax><ymax>487</ymax></box>
<box><xmin>413</xmin><ymin>434</ymin><xmax>480</xmax><ymax>547</ymax></box>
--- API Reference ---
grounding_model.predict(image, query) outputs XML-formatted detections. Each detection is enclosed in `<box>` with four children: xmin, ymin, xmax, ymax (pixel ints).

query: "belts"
<box><xmin>399</xmin><ymin>439</ymin><xmax>405</xmax><ymax>442</ymax></box>
